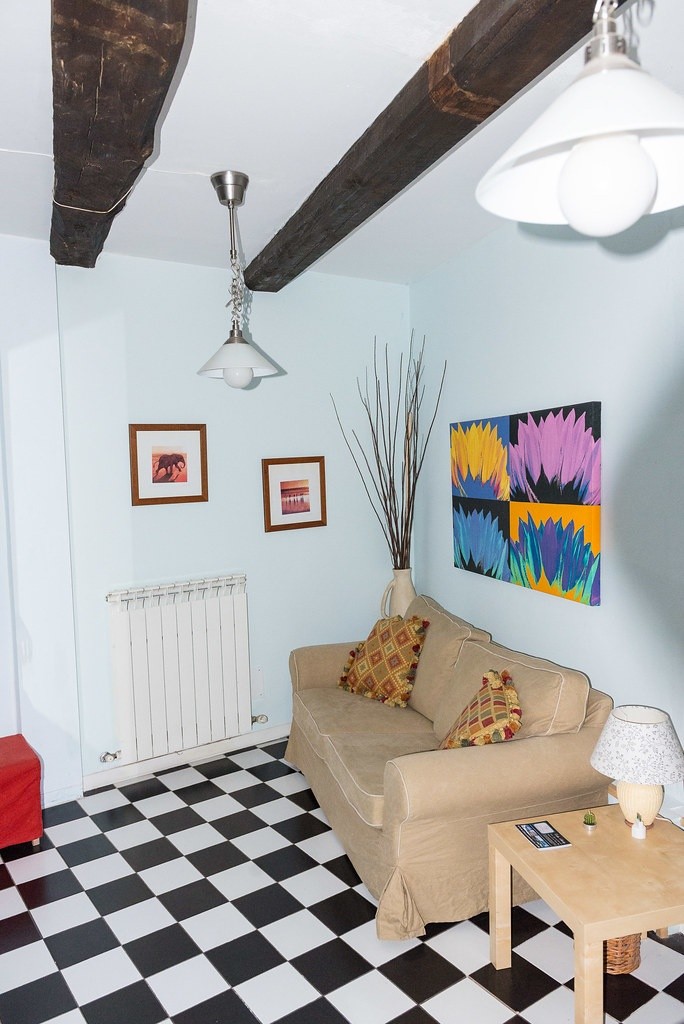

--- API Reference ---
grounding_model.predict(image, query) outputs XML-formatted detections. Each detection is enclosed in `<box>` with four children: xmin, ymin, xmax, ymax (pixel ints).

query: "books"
<box><xmin>515</xmin><ymin>821</ymin><xmax>572</xmax><ymax>851</ymax></box>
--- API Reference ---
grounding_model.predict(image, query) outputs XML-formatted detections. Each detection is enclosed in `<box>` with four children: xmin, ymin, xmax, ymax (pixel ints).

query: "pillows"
<box><xmin>437</xmin><ymin>667</ymin><xmax>524</xmax><ymax>749</ymax></box>
<box><xmin>337</xmin><ymin>614</ymin><xmax>431</xmax><ymax>708</ymax></box>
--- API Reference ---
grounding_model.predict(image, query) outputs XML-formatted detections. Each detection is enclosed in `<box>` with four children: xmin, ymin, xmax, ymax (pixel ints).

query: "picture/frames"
<box><xmin>128</xmin><ymin>422</ymin><xmax>209</xmax><ymax>507</ymax></box>
<box><xmin>262</xmin><ymin>454</ymin><xmax>327</xmax><ymax>533</ymax></box>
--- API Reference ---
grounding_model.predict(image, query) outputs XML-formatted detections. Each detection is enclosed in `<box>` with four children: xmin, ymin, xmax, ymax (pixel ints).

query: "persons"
<box><xmin>284</xmin><ymin>492</ymin><xmax>305</xmax><ymax>508</ymax></box>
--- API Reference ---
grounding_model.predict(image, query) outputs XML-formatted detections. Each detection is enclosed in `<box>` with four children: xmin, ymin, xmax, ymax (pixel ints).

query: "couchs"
<box><xmin>283</xmin><ymin>594</ymin><xmax>613</xmax><ymax>940</ymax></box>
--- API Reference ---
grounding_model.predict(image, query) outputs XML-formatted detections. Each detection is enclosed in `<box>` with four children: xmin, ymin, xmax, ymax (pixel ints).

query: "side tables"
<box><xmin>488</xmin><ymin>802</ymin><xmax>684</xmax><ymax>1023</ymax></box>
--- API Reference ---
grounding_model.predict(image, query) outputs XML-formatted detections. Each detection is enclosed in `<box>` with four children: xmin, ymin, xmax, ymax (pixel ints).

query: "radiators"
<box><xmin>99</xmin><ymin>574</ymin><xmax>269</xmax><ymax>779</ymax></box>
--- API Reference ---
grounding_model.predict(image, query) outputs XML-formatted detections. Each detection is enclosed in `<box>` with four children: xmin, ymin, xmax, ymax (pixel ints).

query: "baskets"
<box><xmin>603</xmin><ymin>932</ymin><xmax>642</xmax><ymax>976</ymax></box>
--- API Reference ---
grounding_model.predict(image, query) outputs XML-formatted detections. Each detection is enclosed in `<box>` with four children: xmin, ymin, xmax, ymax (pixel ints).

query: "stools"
<box><xmin>0</xmin><ymin>734</ymin><xmax>42</xmax><ymax>850</ymax></box>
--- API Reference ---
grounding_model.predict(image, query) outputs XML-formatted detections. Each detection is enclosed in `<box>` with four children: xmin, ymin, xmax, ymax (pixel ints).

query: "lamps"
<box><xmin>474</xmin><ymin>0</ymin><xmax>684</xmax><ymax>236</ymax></box>
<box><xmin>197</xmin><ymin>171</ymin><xmax>277</xmax><ymax>387</ymax></box>
<box><xmin>590</xmin><ymin>705</ymin><xmax>684</xmax><ymax>831</ymax></box>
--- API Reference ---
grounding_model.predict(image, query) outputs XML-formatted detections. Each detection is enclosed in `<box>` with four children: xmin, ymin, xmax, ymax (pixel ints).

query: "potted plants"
<box><xmin>330</xmin><ymin>329</ymin><xmax>447</xmax><ymax>620</ymax></box>
